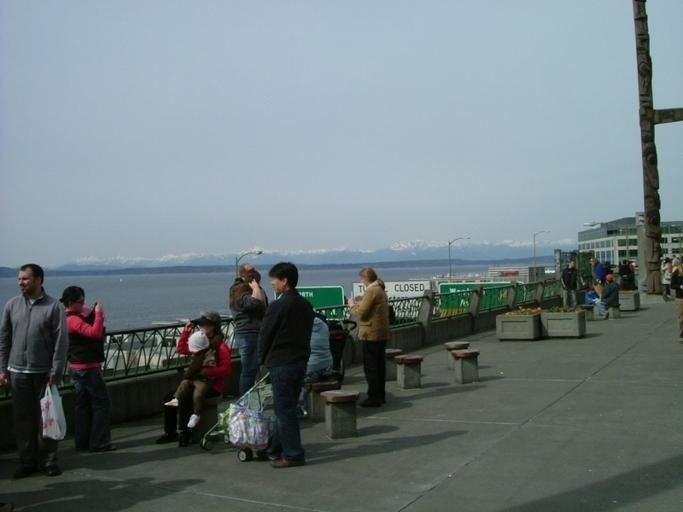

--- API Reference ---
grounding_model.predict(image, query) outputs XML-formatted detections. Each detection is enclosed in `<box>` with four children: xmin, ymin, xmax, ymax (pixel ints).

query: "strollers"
<box><xmin>199</xmin><ymin>370</ymin><xmax>272</xmax><ymax>461</ymax></box>
<box><xmin>295</xmin><ymin>320</ymin><xmax>358</xmax><ymax>416</ymax></box>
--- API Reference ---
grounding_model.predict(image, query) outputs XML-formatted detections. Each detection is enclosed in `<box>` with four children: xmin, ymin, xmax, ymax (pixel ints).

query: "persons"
<box><xmin>0</xmin><ymin>263</ymin><xmax>70</xmax><ymax>479</ymax></box>
<box><xmin>349</xmin><ymin>267</ymin><xmax>390</xmax><ymax>409</ymax></box>
<box><xmin>560</xmin><ymin>255</ymin><xmax>683</xmax><ymax>344</ymax></box>
<box><xmin>155</xmin><ymin>261</ymin><xmax>334</xmax><ymax>467</ymax></box>
<box><xmin>58</xmin><ymin>286</ymin><xmax>117</xmax><ymax>452</ymax></box>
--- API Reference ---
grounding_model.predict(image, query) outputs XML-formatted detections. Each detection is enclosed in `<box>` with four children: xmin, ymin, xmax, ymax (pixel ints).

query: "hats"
<box><xmin>606</xmin><ymin>274</ymin><xmax>613</xmax><ymax>282</ymax></box>
<box><xmin>187</xmin><ymin>330</ymin><xmax>211</xmax><ymax>353</ymax></box>
<box><xmin>190</xmin><ymin>309</ymin><xmax>222</xmax><ymax>328</ymax></box>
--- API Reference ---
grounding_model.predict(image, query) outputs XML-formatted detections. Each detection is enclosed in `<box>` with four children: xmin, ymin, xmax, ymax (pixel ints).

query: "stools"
<box><xmin>450</xmin><ymin>348</ymin><xmax>480</xmax><ymax>385</ymax></box>
<box><xmin>319</xmin><ymin>390</ymin><xmax>360</xmax><ymax>442</ymax></box>
<box><xmin>393</xmin><ymin>353</ymin><xmax>424</xmax><ymax>390</ymax></box>
<box><xmin>443</xmin><ymin>340</ymin><xmax>470</xmax><ymax>371</ymax></box>
<box><xmin>384</xmin><ymin>348</ymin><xmax>402</xmax><ymax>382</ymax></box>
<box><xmin>179</xmin><ymin>394</ymin><xmax>226</xmax><ymax>442</ymax></box>
<box><xmin>305</xmin><ymin>380</ymin><xmax>343</xmax><ymax>424</ymax></box>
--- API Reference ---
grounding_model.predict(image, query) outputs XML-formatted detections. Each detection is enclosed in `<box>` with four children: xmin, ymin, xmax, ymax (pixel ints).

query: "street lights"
<box><xmin>234</xmin><ymin>249</ymin><xmax>264</xmax><ymax>279</ymax></box>
<box><xmin>668</xmin><ymin>223</ymin><xmax>682</xmax><ymax>254</ymax></box>
<box><xmin>532</xmin><ymin>229</ymin><xmax>551</xmax><ymax>267</ymax></box>
<box><xmin>580</xmin><ymin>220</ymin><xmax>629</xmax><ymax>264</ymax></box>
<box><xmin>447</xmin><ymin>236</ymin><xmax>473</xmax><ymax>280</ymax></box>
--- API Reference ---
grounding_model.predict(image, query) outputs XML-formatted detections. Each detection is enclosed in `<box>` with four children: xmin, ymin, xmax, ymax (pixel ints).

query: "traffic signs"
<box><xmin>349</xmin><ymin>278</ymin><xmax>434</xmax><ymax>318</ymax></box>
<box><xmin>437</xmin><ymin>280</ymin><xmax>526</xmax><ymax>318</ymax></box>
<box><xmin>272</xmin><ymin>285</ymin><xmax>346</xmax><ymax>321</ymax></box>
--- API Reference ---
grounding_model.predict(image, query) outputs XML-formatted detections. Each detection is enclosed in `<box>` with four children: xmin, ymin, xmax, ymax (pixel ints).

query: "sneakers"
<box><xmin>271</xmin><ymin>458</ymin><xmax>306</xmax><ymax>469</ymax></box>
<box><xmin>187</xmin><ymin>413</ymin><xmax>200</xmax><ymax>428</ymax></box>
<box><xmin>155</xmin><ymin>431</ymin><xmax>178</xmax><ymax>444</ymax></box>
<box><xmin>164</xmin><ymin>397</ymin><xmax>180</xmax><ymax>407</ymax></box>
<box><xmin>90</xmin><ymin>443</ymin><xmax>122</xmax><ymax>452</ymax></box>
<box><xmin>180</xmin><ymin>431</ymin><xmax>193</xmax><ymax>448</ymax></box>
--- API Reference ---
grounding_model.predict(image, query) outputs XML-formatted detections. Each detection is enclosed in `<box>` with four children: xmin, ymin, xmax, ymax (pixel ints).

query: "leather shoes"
<box><xmin>14</xmin><ymin>462</ymin><xmax>37</xmax><ymax>478</ymax></box>
<box><xmin>40</xmin><ymin>461</ymin><xmax>61</xmax><ymax>477</ymax></box>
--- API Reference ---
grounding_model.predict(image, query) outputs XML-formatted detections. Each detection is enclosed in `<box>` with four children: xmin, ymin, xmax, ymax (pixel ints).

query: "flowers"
<box><xmin>503</xmin><ymin>305</ymin><xmax>541</xmax><ymax>315</ymax></box>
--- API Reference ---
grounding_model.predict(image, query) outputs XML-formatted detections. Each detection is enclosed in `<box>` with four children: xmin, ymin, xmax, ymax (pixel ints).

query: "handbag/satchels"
<box><xmin>228</xmin><ymin>402</ymin><xmax>278</xmax><ymax>451</ymax></box>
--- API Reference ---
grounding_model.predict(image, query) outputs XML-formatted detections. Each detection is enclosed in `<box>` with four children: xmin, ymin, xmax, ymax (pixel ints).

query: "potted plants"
<box><xmin>539</xmin><ymin>303</ymin><xmax>587</xmax><ymax>338</ymax></box>
<box><xmin>617</xmin><ymin>289</ymin><xmax>641</xmax><ymax>313</ymax></box>
